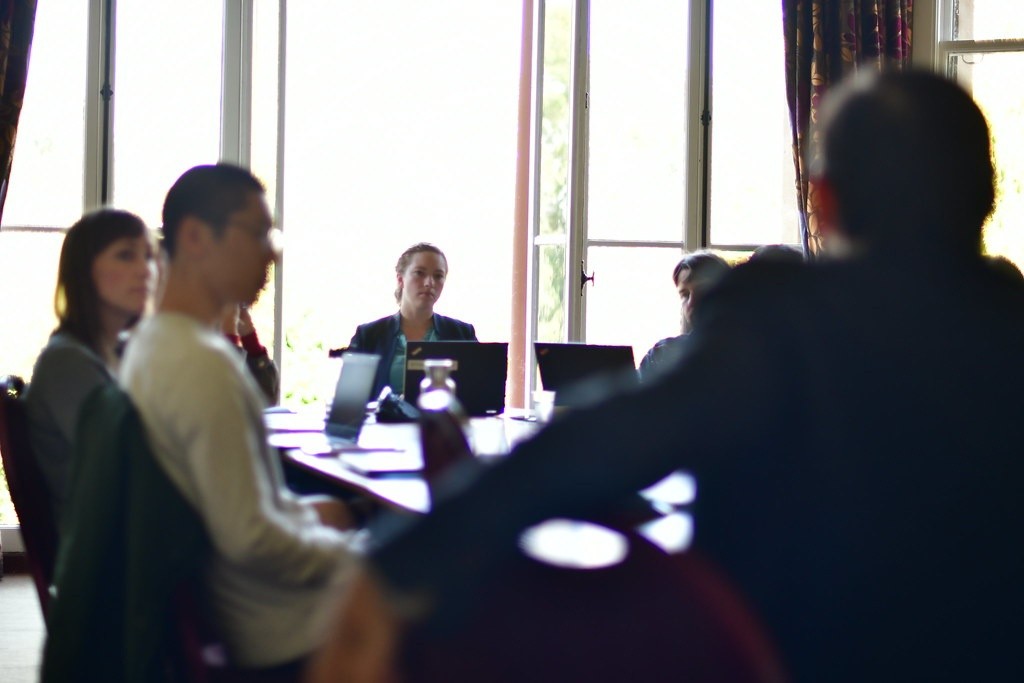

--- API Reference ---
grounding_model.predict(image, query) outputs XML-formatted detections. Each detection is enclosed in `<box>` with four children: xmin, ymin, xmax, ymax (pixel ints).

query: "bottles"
<box><xmin>419</xmin><ymin>358</ymin><xmax>456</xmax><ymax>471</ymax></box>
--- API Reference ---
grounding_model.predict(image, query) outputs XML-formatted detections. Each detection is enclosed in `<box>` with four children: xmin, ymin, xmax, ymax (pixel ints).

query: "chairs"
<box><xmin>0</xmin><ymin>375</ymin><xmax>301</xmax><ymax>683</ymax></box>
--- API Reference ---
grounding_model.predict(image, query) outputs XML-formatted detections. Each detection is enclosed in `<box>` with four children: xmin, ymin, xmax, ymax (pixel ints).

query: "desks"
<box><xmin>262</xmin><ymin>410</ymin><xmax>696</xmax><ymax>683</ymax></box>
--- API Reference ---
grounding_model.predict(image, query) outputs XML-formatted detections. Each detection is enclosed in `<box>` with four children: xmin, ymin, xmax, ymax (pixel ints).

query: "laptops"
<box><xmin>535</xmin><ymin>341</ymin><xmax>634</xmax><ymax>393</ymax></box>
<box><xmin>404</xmin><ymin>339</ymin><xmax>508</xmax><ymax>417</ymax></box>
<box><xmin>268</xmin><ymin>352</ymin><xmax>382</xmax><ymax>447</ymax></box>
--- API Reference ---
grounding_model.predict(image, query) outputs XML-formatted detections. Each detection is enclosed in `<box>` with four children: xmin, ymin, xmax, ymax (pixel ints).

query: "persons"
<box><xmin>348</xmin><ymin>242</ymin><xmax>480</xmax><ymax>404</ymax></box>
<box><xmin>318</xmin><ymin>56</ymin><xmax>1024</xmax><ymax>683</ymax></box>
<box><xmin>639</xmin><ymin>253</ymin><xmax>727</xmax><ymax>373</ymax></box>
<box><xmin>23</xmin><ymin>161</ymin><xmax>373</xmax><ymax>683</ymax></box>
<box><xmin>748</xmin><ymin>244</ymin><xmax>804</xmax><ymax>262</ymax></box>
